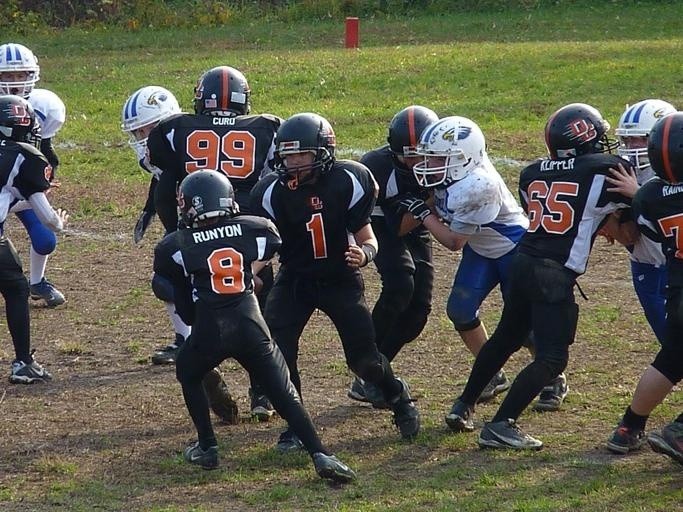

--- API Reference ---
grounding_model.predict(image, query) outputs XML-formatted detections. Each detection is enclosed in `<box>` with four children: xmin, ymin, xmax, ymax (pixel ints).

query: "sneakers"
<box><xmin>479</xmin><ymin>371</ymin><xmax>510</xmax><ymax>398</ymax></box>
<box><xmin>534</xmin><ymin>372</ymin><xmax>568</xmax><ymax>410</ymax></box>
<box><xmin>203</xmin><ymin>371</ymin><xmax>237</xmax><ymax>421</ymax></box>
<box><xmin>347</xmin><ymin>377</ymin><xmax>420</xmax><ymax>438</ymax></box>
<box><xmin>248</xmin><ymin>387</ymin><xmax>276</xmax><ymax>421</ymax></box>
<box><xmin>276</xmin><ymin>430</ymin><xmax>304</xmax><ymax>452</ymax></box>
<box><xmin>152</xmin><ymin>343</ymin><xmax>181</xmax><ymax>365</ymax></box>
<box><xmin>9</xmin><ymin>355</ymin><xmax>53</xmax><ymax>384</ymax></box>
<box><xmin>183</xmin><ymin>442</ymin><xmax>219</xmax><ymax>471</ymax></box>
<box><xmin>648</xmin><ymin>422</ymin><xmax>683</xmax><ymax>466</ymax></box>
<box><xmin>445</xmin><ymin>399</ymin><xmax>474</xmax><ymax>432</ymax></box>
<box><xmin>29</xmin><ymin>277</ymin><xmax>65</xmax><ymax>305</ymax></box>
<box><xmin>607</xmin><ymin>422</ymin><xmax>644</xmax><ymax>452</ymax></box>
<box><xmin>478</xmin><ymin>420</ymin><xmax>544</xmax><ymax>450</ymax></box>
<box><xmin>312</xmin><ymin>453</ymin><xmax>356</xmax><ymax>482</ymax></box>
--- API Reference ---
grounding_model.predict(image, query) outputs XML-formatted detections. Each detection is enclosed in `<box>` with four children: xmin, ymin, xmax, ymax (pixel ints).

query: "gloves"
<box><xmin>400</xmin><ymin>196</ymin><xmax>431</xmax><ymax>223</ymax></box>
<box><xmin>134</xmin><ymin>211</ymin><xmax>152</xmax><ymax>244</ymax></box>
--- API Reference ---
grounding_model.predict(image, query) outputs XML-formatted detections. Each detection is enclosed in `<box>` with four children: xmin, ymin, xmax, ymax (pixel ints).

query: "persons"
<box><xmin>447</xmin><ymin>99</ymin><xmax>683</xmax><ymax>465</ymax></box>
<box><xmin>119</xmin><ymin>67</ymin><xmax>283</xmax><ymax>423</ymax></box>
<box><xmin>0</xmin><ymin>41</ymin><xmax>67</xmax><ymax>307</ymax></box>
<box><xmin>154</xmin><ymin>169</ymin><xmax>358</xmax><ymax>484</ymax></box>
<box><xmin>235</xmin><ymin>112</ymin><xmax>420</xmax><ymax>452</ymax></box>
<box><xmin>348</xmin><ymin>104</ymin><xmax>442</xmax><ymax>403</ymax></box>
<box><xmin>395</xmin><ymin>114</ymin><xmax>569</xmax><ymax>411</ymax></box>
<box><xmin>0</xmin><ymin>95</ymin><xmax>67</xmax><ymax>385</ymax></box>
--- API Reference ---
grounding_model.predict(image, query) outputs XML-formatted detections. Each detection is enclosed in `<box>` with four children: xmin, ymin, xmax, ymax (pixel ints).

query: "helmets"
<box><xmin>0</xmin><ymin>43</ymin><xmax>41</xmax><ymax>99</ymax></box>
<box><xmin>120</xmin><ymin>86</ymin><xmax>180</xmax><ymax>160</ymax></box>
<box><xmin>194</xmin><ymin>65</ymin><xmax>250</xmax><ymax>117</ymax></box>
<box><xmin>273</xmin><ymin>112</ymin><xmax>337</xmax><ymax>189</ymax></box>
<box><xmin>545</xmin><ymin>103</ymin><xmax>620</xmax><ymax>161</ymax></box>
<box><xmin>614</xmin><ymin>99</ymin><xmax>678</xmax><ymax>169</ymax></box>
<box><xmin>412</xmin><ymin>116</ymin><xmax>485</xmax><ymax>190</ymax></box>
<box><xmin>647</xmin><ymin>112</ymin><xmax>683</xmax><ymax>186</ymax></box>
<box><xmin>0</xmin><ymin>95</ymin><xmax>42</xmax><ymax>148</ymax></box>
<box><xmin>387</xmin><ymin>105</ymin><xmax>439</xmax><ymax>177</ymax></box>
<box><xmin>177</xmin><ymin>169</ymin><xmax>240</xmax><ymax>229</ymax></box>
